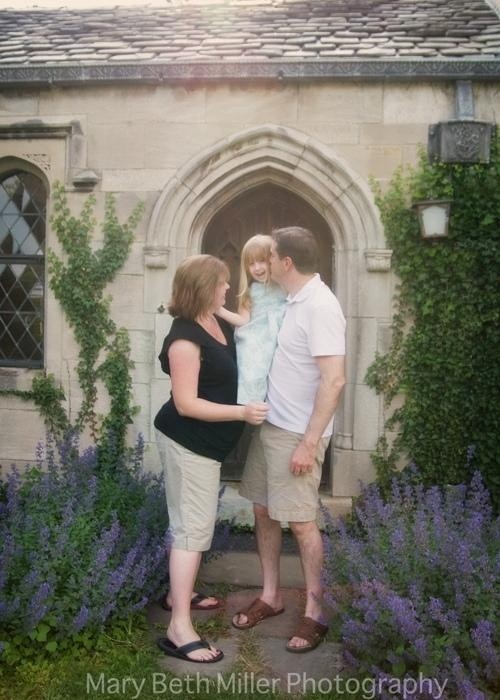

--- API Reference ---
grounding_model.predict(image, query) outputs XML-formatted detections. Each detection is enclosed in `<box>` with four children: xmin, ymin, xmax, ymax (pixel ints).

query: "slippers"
<box><xmin>156</xmin><ymin>638</ymin><xmax>224</xmax><ymax>664</ymax></box>
<box><xmin>161</xmin><ymin>593</ymin><xmax>224</xmax><ymax>611</ymax></box>
<box><xmin>232</xmin><ymin>597</ymin><xmax>285</xmax><ymax>629</ymax></box>
<box><xmin>286</xmin><ymin>616</ymin><xmax>329</xmax><ymax>653</ymax></box>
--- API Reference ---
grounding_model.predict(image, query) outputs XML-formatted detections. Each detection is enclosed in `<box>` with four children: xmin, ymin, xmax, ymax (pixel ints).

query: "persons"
<box><xmin>153</xmin><ymin>251</ymin><xmax>270</xmax><ymax>664</ymax></box>
<box><xmin>212</xmin><ymin>233</ymin><xmax>287</xmax><ymax>406</ymax></box>
<box><xmin>227</xmin><ymin>226</ymin><xmax>348</xmax><ymax>654</ymax></box>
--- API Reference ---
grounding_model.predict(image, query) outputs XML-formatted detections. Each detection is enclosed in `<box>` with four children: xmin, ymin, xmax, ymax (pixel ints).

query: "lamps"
<box><xmin>411</xmin><ymin>196</ymin><xmax>455</xmax><ymax>248</ymax></box>
